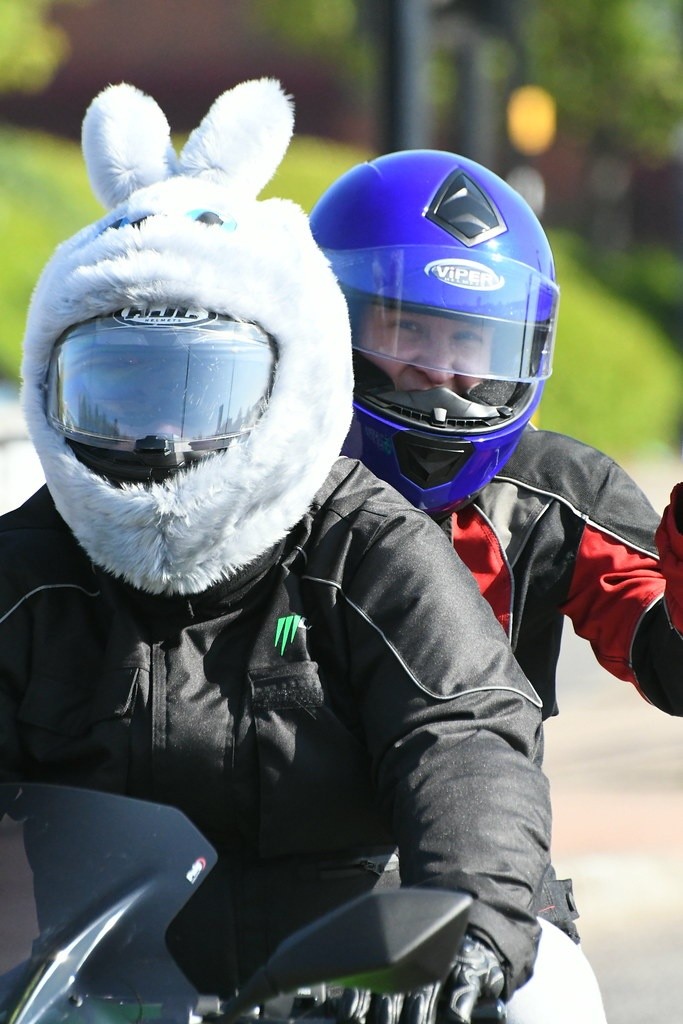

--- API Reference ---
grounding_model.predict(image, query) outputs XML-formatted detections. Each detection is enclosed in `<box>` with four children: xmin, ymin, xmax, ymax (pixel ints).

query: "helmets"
<box><xmin>310</xmin><ymin>150</ymin><xmax>559</xmax><ymax>523</ymax></box>
<box><xmin>20</xmin><ymin>182</ymin><xmax>353</xmax><ymax>595</ymax></box>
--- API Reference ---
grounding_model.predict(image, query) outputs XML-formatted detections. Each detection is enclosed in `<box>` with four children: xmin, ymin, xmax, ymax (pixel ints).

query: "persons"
<box><xmin>0</xmin><ymin>233</ymin><xmax>610</xmax><ymax>1024</ymax></box>
<box><xmin>296</xmin><ymin>148</ymin><xmax>683</xmax><ymax>724</ymax></box>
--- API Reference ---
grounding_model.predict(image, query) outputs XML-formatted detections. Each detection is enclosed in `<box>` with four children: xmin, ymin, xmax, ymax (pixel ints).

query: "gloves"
<box><xmin>655</xmin><ymin>482</ymin><xmax>683</xmax><ymax>635</ymax></box>
<box><xmin>337</xmin><ymin>932</ymin><xmax>509</xmax><ymax>1024</ymax></box>
